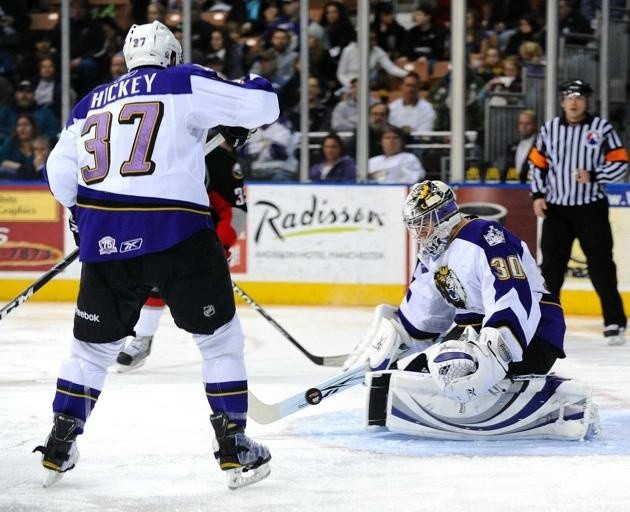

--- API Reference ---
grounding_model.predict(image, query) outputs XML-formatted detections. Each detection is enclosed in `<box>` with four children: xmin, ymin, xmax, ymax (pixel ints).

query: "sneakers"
<box><xmin>210</xmin><ymin>411</ymin><xmax>271</xmax><ymax>469</ymax></box>
<box><xmin>604</xmin><ymin>323</ymin><xmax>619</xmax><ymax>337</ymax></box>
<box><xmin>43</xmin><ymin>411</ymin><xmax>79</xmax><ymax>473</ymax></box>
<box><xmin>117</xmin><ymin>334</ymin><xmax>153</xmax><ymax>365</ymax></box>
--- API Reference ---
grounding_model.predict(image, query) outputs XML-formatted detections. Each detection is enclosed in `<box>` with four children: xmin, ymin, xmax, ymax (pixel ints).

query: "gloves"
<box><xmin>68</xmin><ymin>214</ymin><xmax>80</xmax><ymax>247</ymax></box>
<box><xmin>216</xmin><ymin>124</ymin><xmax>258</xmax><ymax>149</ymax></box>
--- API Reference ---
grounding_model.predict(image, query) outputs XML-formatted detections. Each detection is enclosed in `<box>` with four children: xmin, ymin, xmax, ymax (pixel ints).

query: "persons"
<box><xmin>116</xmin><ymin>123</ymin><xmax>260</xmax><ymax>367</ymax></box>
<box><xmin>338</xmin><ymin>179</ymin><xmax>605</xmax><ymax>447</ymax></box>
<box><xmin>526</xmin><ymin>77</ymin><xmax>630</xmax><ymax>344</ymax></box>
<box><xmin>36</xmin><ymin>19</ymin><xmax>284</xmax><ymax>474</ymax></box>
<box><xmin>2</xmin><ymin>0</ymin><xmax>603</xmax><ymax>187</ymax></box>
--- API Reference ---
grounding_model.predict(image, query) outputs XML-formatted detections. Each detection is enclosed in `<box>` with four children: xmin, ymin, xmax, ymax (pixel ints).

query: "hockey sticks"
<box><xmin>248</xmin><ymin>324</ymin><xmax>457</xmax><ymax>424</ymax></box>
<box><xmin>230</xmin><ymin>281</ymin><xmax>351</xmax><ymax>365</ymax></box>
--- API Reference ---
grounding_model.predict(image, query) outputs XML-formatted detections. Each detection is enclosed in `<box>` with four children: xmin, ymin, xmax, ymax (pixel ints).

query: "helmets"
<box><xmin>558</xmin><ymin>80</ymin><xmax>593</xmax><ymax>98</ymax></box>
<box><xmin>123</xmin><ymin>19</ymin><xmax>183</xmax><ymax>72</ymax></box>
<box><xmin>404</xmin><ymin>180</ymin><xmax>460</xmax><ymax>257</ymax></box>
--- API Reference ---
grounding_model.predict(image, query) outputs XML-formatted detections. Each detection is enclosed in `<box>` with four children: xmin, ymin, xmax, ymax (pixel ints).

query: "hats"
<box><xmin>17</xmin><ymin>80</ymin><xmax>33</xmax><ymax>91</ymax></box>
